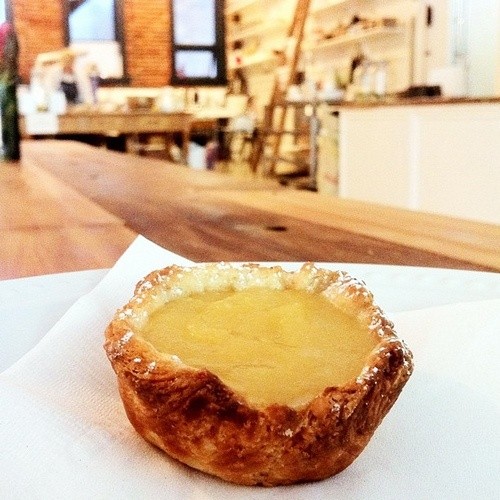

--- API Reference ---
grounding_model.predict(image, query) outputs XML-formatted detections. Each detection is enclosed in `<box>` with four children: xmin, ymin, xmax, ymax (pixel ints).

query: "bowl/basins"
<box><xmin>128</xmin><ymin>96</ymin><xmax>155</xmax><ymax>109</ymax></box>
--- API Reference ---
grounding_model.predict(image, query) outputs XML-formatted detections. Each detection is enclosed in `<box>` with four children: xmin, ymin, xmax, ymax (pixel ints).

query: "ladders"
<box><xmin>251</xmin><ymin>1</ymin><xmax>308</xmax><ymax>172</ymax></box>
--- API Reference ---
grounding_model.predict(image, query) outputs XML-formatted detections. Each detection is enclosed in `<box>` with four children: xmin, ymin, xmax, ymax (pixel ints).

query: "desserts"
<box><xmin>105</xmin><ymin>260</ymin><xmax>414</xmax><ymax>485</ymax></box>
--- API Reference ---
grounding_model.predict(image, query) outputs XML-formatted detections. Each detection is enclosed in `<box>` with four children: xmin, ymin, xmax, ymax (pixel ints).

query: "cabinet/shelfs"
<box><xmin>308</xmin><ymin>98</ymin><xmax>500</xmax><ymax>225</ymax></box>
<box><xmin>226</xmin><ymin>0</ymin><xmax>451</xmax><ymax>189</ymax></box>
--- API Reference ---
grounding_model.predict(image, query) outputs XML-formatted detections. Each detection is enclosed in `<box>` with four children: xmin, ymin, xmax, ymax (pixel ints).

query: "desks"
<box><xmin>0</xmin><ymin>139</ymin><xmax>500</xmax><ymax>499</ymax></box>
<box><xmin>56</xmin><ymin>109</ymin><xmax>231</xmax><ymax>166</ymax></box>
